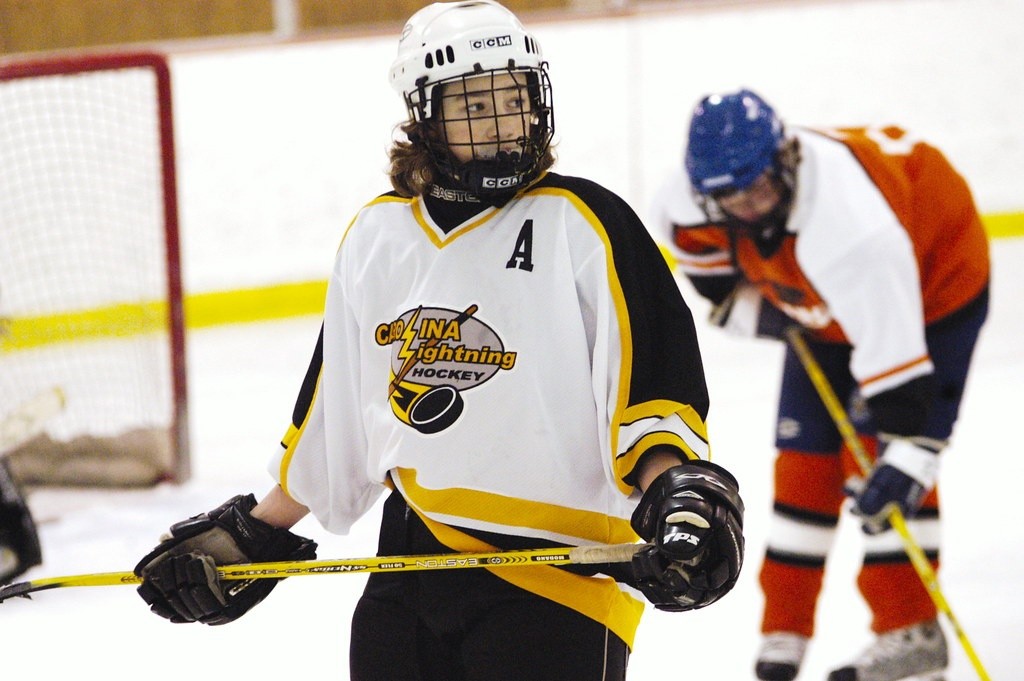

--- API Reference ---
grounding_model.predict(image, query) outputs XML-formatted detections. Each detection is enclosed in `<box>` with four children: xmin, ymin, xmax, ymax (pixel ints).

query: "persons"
<box><xmin>665</xmin><ymin>87</ymin><xmax>990</xmax><ymax>681</ymax></box>
<box><xmin>133</xmin><ymin>1</ymin><xmax>746</xmax><ymax>681</ymax></box>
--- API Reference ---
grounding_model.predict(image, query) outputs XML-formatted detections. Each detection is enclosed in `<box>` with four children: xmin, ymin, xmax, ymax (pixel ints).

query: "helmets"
<box><xmin>685</xmin><ymin>89</ymin><xmax>784</xmax><ymax>197</ymax></box>
<box><xmin>390</xmin><ymin>0</ymin><xmax>554</xmax><ymax>208</ymax></box>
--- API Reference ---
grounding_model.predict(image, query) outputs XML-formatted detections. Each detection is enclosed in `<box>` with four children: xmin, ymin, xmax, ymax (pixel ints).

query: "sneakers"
<box><xmin>756</xmin><ymin>630</ymin><xmax>810</xmax><ymax>681</ymax></box>
<box><xmin>826</xmin><ymin>622</ymin><xmax>949</xmax><ymax>680</ymax></box>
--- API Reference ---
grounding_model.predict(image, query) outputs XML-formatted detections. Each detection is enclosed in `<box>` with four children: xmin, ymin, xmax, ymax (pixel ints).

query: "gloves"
<box><xmin>728</xmin><ymin>294</ymin><xmax>821</xmax><ymax>340</ymax></box>
<box><xmin>136</xmin><ymin>493</ymin><xmax>317</xmax><ymax>624</ymax></box>
<box><xmin>626</xmin><ymin>459</ymin><xmax>744</xmax><ymax>614</ymax></box>
<box><xmin>851</xmin><ymin>439</ymin><xmax>944</xmax><ymax>534</ymax></box>
